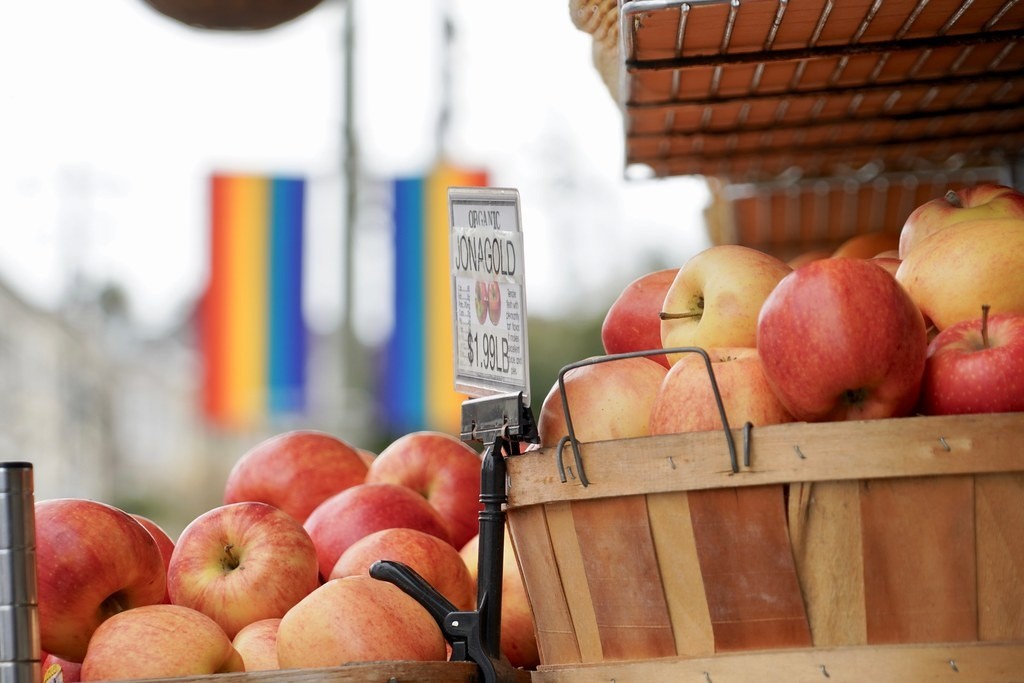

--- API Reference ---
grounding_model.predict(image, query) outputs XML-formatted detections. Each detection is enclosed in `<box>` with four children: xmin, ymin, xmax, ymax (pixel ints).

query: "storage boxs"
<box><xmin>492</xmin><ymin>411</ymin><xmax>1024</xmax><ymax>683</ymax></box>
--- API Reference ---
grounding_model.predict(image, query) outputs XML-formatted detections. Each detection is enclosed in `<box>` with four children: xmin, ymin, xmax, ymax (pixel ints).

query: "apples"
<box><xmin>533</xmin><ymin>177</ymin><xmax>1024</xmax><ymax>449</ymax></box>
<box><xmin>26</xmin><ymin>426</ymin><xmax>541</xmax><ymax>683</ymax></box>
